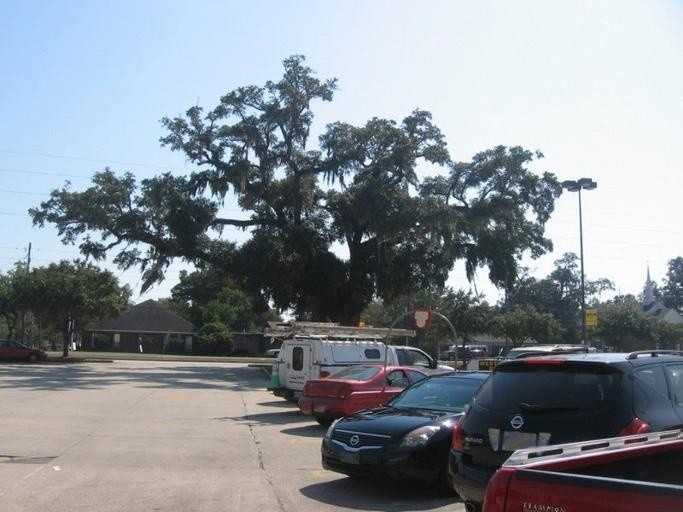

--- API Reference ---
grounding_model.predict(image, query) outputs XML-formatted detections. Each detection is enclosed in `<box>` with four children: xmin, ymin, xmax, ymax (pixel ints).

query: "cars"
<box><xmin>0</xmin><ymin>339</ymin><xmax>48</xmax><ymax>363</ymax></box>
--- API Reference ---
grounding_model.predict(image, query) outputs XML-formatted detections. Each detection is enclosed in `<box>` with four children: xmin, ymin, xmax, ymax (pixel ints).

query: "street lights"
<box><xmin>562</xmin><ymin>177</ymin><xmax>597</xmax><ymax>345</ymax></box>
